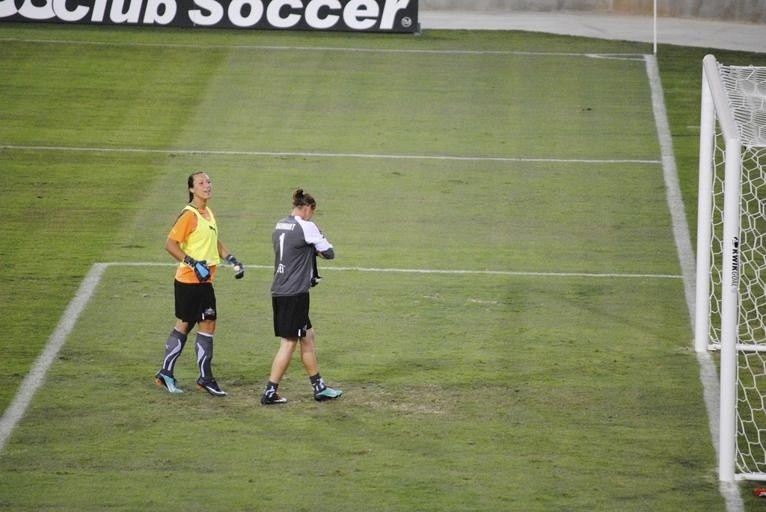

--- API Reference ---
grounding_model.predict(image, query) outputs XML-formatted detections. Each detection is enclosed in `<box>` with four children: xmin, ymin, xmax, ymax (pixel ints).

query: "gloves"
<box><xmin>223</xmin><ymin>253</ymin><xmax>245</xmax><ymax>280</ymax></box>
<box><xmin>183</xmin><ymin>254</ymin><xmax>212</xmax><ymax>282</ymax></box>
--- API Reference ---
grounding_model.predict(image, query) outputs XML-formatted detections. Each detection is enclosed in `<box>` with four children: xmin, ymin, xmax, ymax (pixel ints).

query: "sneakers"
<box><xmin>313</xmin><ymin>386</ymin><xmax>343</xmax><ymax>401</ymax></box>
<box><xmin>153</xmin><ymin>368</ymin><xmax>184</xmax><ymax>394</ymax></box>
<box><xmin>260</xmin><ymin>391</ymin><xmax>287</xmax><ymax>405</ymax></box>
<box><xmin>195</xmin><ymin>376</ymin><xmax>226</xmax><ymax>397</ymax></box>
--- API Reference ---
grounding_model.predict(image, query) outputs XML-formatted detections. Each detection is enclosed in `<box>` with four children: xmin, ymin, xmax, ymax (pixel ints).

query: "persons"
<box><xmin>259</xmin><ymin>189</ymin><xmax>342</xmax><ymax>404</ymax></box>
<box><xmin>152</xmin><ymin>170</ymin><xmax>245</xmax><ymax>398</ymax></box>
